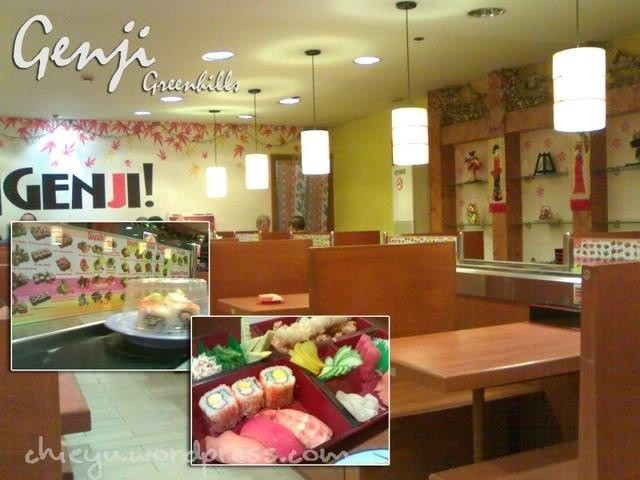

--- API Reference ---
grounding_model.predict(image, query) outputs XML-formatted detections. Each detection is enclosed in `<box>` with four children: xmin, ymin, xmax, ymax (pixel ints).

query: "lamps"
<box><xmin>245</xmin><ymin>88</ymin><xmax>269</xmax><ymax>190</ymax></box>
<box><xmin>552</xmin><ymin>0</ymin><xmax>606</xmax><ymax>133</ymax></box>
<box><xmin>205</xmin><ymin>110</ymin><xmax>227</xmax><ymax>199</ymax></box>
<box><xmin>392</xmin><ymin>1</ymin><xmax>429</xmax><ymax>165</ymax></box>
<box><xmin>300</xmin><ymin>49</ymin><xmax>330</xmax><ymax>175</ymax></box>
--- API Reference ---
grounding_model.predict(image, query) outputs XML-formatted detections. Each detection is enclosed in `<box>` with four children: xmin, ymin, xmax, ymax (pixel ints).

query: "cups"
<box><xmin>555</xmin><ymin>247</ymin><xmax>564</xmax><ymax>264</ymax></box>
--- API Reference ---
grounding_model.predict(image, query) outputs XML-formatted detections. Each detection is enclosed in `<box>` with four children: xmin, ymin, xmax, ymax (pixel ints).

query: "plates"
<box><xmin>103</xmin><ymin>309</ymin><xmax>208</xmax><ymax>351</ymax></box>
<box><xmin>532</xmin><ymin>216</ymin><xmax>562</xmax><ymax>223</ymax></box>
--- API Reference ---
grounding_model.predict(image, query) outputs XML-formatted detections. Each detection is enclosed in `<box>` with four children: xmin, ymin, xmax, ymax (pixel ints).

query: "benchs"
<box><xmin>211</xmin><ymin>238</ymin><xmax>313</xmax><ymax>315</ymax></box>
<box><xmin>309</xmin><ymin>241</ymin><xmax>540</xmax><ymax>480</ymax></box>
<box><xmin>430</xmin><ymin>260</ymin><xmax>640</xmax><ymax>480</ymax></box>
<box><xmin>58</xmin><ymin>372</ymin><xmax>93</xmax><ymax>434</ymax></box>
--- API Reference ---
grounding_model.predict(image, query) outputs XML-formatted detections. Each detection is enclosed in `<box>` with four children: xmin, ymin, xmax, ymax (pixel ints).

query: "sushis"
<box><xmin>259</xmin><ymin>365</ymin><xmax>296</xmax><ymax>408</ymax></box>
<box><xmin>230</xmin><ymin>375</ymin><xmax>263</xmax><ymax>419</ymax></box>
<box><xmin>137</xmin><ymin>292</ymin><xmax>169</xmax><ymax>329</ymax></box>
<box><xmin>197</xmin><ymin>384</ymin><xmax>240</xmax><ymax>436</ymax></box>
<box><xmin>164</xmin><ymin>290</ymin><xmax>200</xmax><ymax>329</ymax></box>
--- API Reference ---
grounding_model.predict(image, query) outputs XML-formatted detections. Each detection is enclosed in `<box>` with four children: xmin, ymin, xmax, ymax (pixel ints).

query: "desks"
<box><xmin>217</xmin><ymin>292</ymin><xmax>310</xmax><ymax>314</ymax></box>
<box><xmin>0</xmin><ymin>243</ymin><xmax>61</xmax><ymax>480</ymax></box>
<box><xmin>390</xmin><ymin>322</ymin><xmax>581</xmax><ymax>463</ymax></box>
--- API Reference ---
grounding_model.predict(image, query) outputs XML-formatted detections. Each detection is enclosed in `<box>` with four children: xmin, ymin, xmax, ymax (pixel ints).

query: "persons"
<box><xmin>490</xmin><ymin>144</ymin><xmax>503</xmax><ymax>202</ymax></box>
<box><xmin>287</xmin><ymin>216</ymin><xmax>305</xmax><ymax>235</ymax></box>
<box><xmin>572</xmin><ymin>131</ymin><xmax>586</xmax><ymax>195</ymax></box>
<box><xmin>256</xmin><ymin>214</ymin><xmax>270</xmax><ymax>233</ymax></box>
